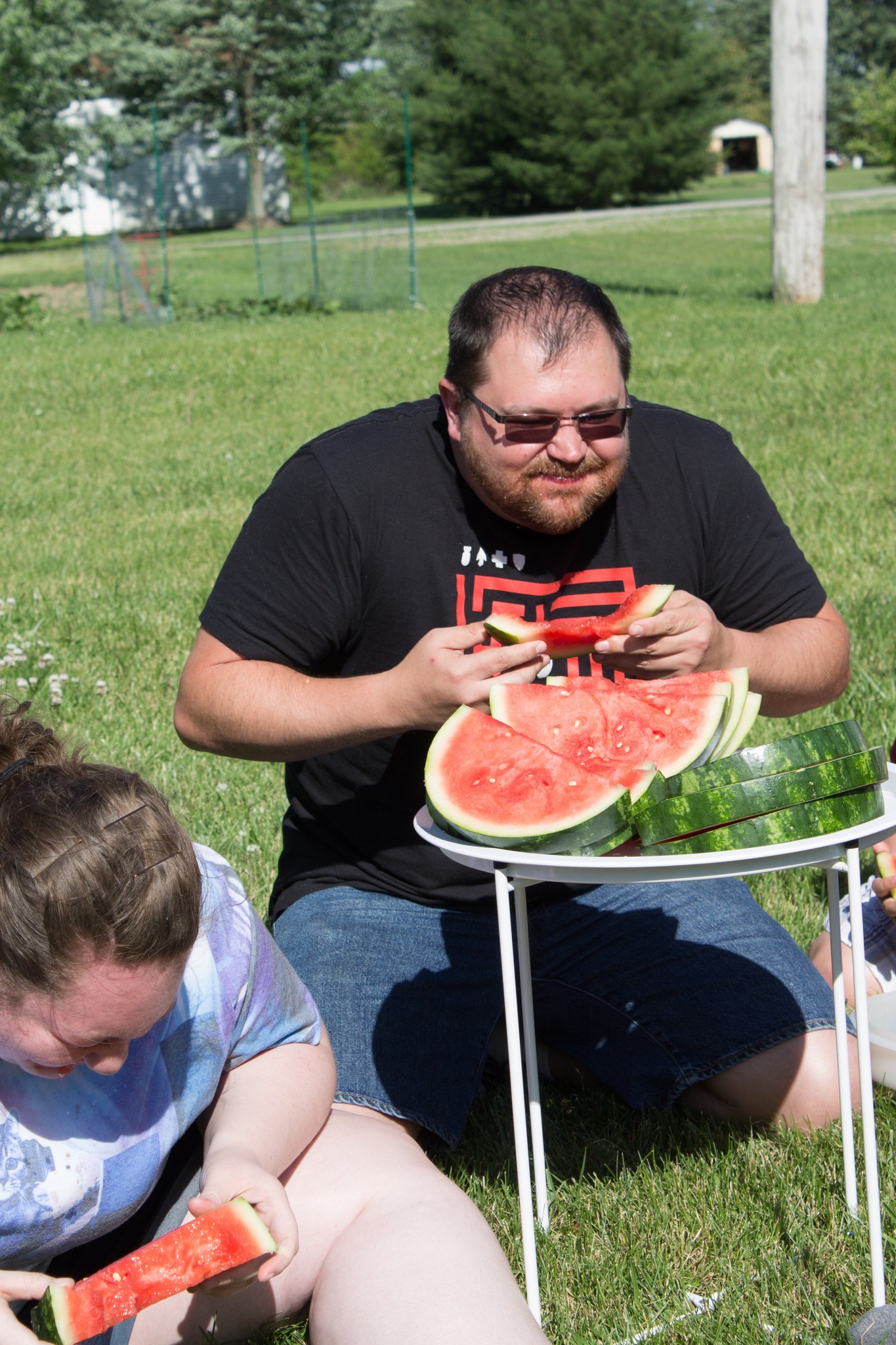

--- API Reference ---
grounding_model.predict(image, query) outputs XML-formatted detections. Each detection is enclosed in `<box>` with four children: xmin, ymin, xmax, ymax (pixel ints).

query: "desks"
<box><xmin>412</xmin><ymin>763</ymin><xmax>896</xmax><ymax>1345</ymax></box>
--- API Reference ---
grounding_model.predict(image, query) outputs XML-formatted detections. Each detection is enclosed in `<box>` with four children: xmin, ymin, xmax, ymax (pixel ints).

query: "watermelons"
<box><xmin>30</xmin><ymin>1196</ymin><xmax>277</xmax><ymax>1345</ymax></box>
<box><xmin>634</xmin><ymin>719</ymin><xmax>889</xmax><ymax>854</ymax></box>
<box><xmin>484</xmin><ymin>584</ymin><xmax>675</xmax><ymax>658</ymax></box>
<box><xmin>876</xmin><ymin>852</ymin><xmax>896</xmax><ymax>899</ymax></box>
<box><xmin>423</xmin><ymin>667</ymin><xmax>762</xmax><ymax>858</ymax></box>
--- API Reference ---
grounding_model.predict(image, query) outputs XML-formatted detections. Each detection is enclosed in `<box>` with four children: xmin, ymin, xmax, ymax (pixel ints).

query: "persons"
<box><xmin>174</xmin><ymin>264</ymin><xmax>896</xmax><ymax>1142</ymax></box>
<box><xmin>0</xmin><ymin>696</ymin><xmax>558</xmax><ymax>1345</ymax></box>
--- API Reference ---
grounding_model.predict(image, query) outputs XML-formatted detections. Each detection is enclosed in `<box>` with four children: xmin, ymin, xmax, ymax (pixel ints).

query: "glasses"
<box><xmin>458</xmin><ymin>379</ymin><xmax>632</xmax><ymax>443</ymax></box>
